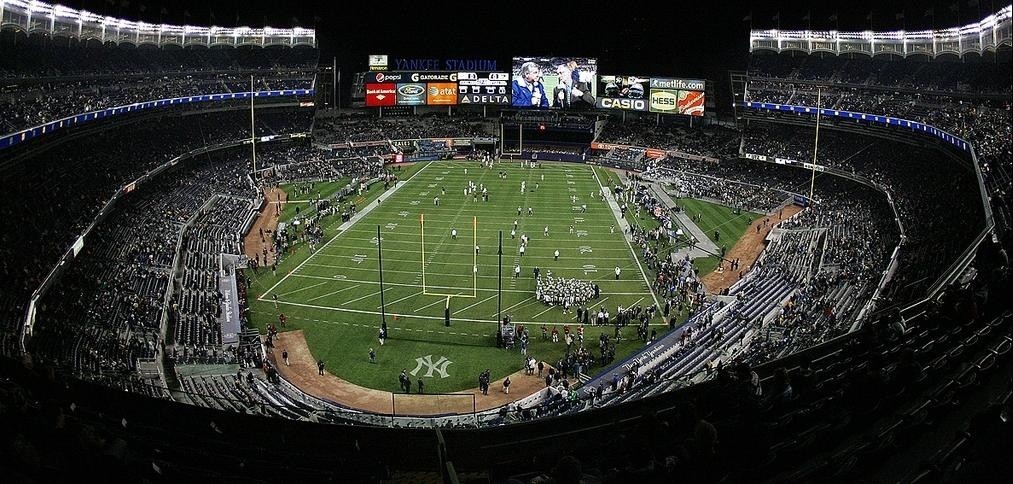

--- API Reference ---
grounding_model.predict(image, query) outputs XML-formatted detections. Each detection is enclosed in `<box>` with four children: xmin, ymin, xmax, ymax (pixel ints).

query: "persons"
<box><xmin>1</xmin><ymin>44</ymin><xmax>1013</xmax><ymax>483</ymax></box>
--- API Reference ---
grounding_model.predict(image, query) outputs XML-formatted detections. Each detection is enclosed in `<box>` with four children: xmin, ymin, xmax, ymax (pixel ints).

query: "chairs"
<box><xmin>0</xmin><ymin>46</ymin><xmax>1013</xmax><ymax>484</ymax></box>
